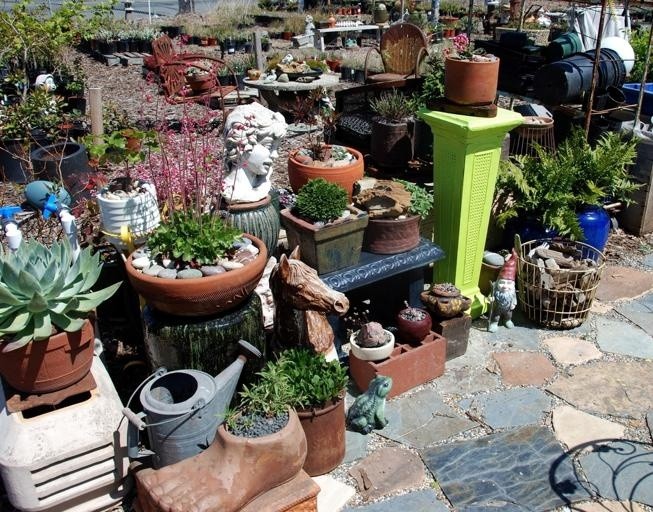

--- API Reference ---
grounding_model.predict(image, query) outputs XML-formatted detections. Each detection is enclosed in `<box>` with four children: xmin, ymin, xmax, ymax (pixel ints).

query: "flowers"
<box><xmin>450</xmin><ymin>32</ymin><xmax>482</xmax><ymax>61</ymax></box>
<box><xmin>127</xmin><ymin>33</ymin><xmax>258</xmax><ymax>266</ymax></box>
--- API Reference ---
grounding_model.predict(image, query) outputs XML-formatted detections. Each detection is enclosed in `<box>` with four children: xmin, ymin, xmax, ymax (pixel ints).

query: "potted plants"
<box><xmin>141</xmin><ymin>358</ymin><xmax>320</xmax><ymax>512</ymax></box>
<box><xmin>287</xmin><ymin>85</ymin><xmax>364</xmax><ymax>202</ymax></box>
<box><xmin>495</xmin><ymin>126</ymin><xmax>648</xmax><ymax>265</ymax></box>
<box><xmin>398</xmin><ymin>307</ymin><xmax>432</xmax><ymax>344</ymax></box>
<box><xmin>420</xmin><ymin>282</ymin><xmax>472</xmax><ymax>319</ymax></box>
<box><xmin>0</xmin><ymin>234</ymin><xmax>124</xmax><ymax>395</ymax></box>
<box><xmin>280</xmin><ymin>177</ymin><xmax>369</xmax><ymax>275</ymax></box>
<box><xmin>355</xmin><ymin>178</ymin><xmax>434</xmax><ymax>255</ymax></box>
<box><xmin>368</xmin><ymin>85</ymin><xmax>418</xmax><ymax>165</ymax></box>
<box><xmin>84</xmin><ymin>120</ymin><xmax>161</xmax><ymax>248</ymax></box>
<box><xmin>283</xmin><ymin>346</ymin><xmax>349</xmax><ymax>477</ymax></box>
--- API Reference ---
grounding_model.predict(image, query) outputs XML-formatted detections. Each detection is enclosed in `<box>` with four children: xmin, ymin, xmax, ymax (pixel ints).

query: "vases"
<box><xmin>126</xmin><ymin>232</ymin><xmax>268</xmax><ymax>319</ymax></box>
<box><xmin>443</xmin><ymin>53</ymin><xmax>499</xmax><ymax>106</ymax></box>
